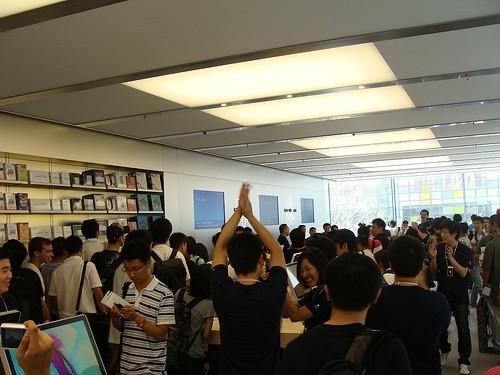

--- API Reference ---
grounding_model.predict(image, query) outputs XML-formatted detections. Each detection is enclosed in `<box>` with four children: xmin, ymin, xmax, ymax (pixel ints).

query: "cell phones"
<box><xmin>114</xmin><ymin>303</ymin><xmax>124</xmax><ymax>309</ymax></box>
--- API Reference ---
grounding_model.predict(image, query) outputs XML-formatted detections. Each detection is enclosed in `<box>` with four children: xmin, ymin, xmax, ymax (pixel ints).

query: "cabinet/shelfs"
<box><xmin>0</xmin><ymin>150</ymin><xmax>165</xmax><ymax>255</ymax></box>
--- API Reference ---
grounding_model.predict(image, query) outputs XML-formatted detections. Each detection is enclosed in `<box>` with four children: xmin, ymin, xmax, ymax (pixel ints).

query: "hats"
<box><xmin>106</xmin><ymin>222</ymin><xmax>130</xmax><ymax>236</ymax></box>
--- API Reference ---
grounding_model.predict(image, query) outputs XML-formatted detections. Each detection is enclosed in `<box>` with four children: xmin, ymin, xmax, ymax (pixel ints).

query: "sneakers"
<box><xmin>440</xmin><ymin>352</ymin><xmax>449</xmax><ymax>365</ymax></box>
<box><xmin>459</xmin><ymin>364</ymin><xmax>470</xmax><ymax>374</ymax></box>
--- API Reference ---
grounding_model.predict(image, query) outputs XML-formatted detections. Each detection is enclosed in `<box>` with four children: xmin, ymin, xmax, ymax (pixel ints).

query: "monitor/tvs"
<box><xmin>0</xmin><ymin>314</ymin><xmax>107</xmax><ymax>375</ymax></box>
<box><xmin>285</xmin><ymin>253</ymin><xmax>302</xmax><ymax>289</ymax></box>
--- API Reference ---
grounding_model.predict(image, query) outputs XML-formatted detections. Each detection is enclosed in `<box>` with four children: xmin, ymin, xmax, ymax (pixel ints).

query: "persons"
<box><xmin>0</xmin><ymin>182</ymin><xmax>500</xmax><ymax>375</ymax></box>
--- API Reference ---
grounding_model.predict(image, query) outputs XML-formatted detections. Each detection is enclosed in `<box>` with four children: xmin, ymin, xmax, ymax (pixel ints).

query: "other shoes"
<box><xmin>470</xmin><ymin>302</ymin><xmax>476</xmax><ymax>308</ymax></box>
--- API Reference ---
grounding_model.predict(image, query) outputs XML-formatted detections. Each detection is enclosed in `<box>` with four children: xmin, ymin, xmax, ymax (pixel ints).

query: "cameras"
<box><xmin>0</xmin><ymin>322</ymin><xmax>28</xmax><ymax>350</ymax></box>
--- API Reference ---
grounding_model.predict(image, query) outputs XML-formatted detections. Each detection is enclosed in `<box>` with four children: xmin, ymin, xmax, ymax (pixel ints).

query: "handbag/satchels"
<box><xmin>318</xmin><ymin>328</ymin><xmax>384</xmax><ymax>375</ymax></box>
<box><xmin>76</xmin><ymin>311</ymin><xmax>111</xmax><ymax>350</ymax></box>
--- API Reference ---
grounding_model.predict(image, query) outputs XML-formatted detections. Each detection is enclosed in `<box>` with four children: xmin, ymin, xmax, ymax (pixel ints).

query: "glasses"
<box><xmin>120</xmin><ymin>263</ymin><xmax>145</xmax><ymax>273</ymax></box>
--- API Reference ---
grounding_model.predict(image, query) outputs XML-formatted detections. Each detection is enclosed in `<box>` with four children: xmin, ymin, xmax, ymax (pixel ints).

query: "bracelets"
<box><xmin>137</xmin><ymin>319</ymin><xmax>146</xmax><ymax>328</ymax></box>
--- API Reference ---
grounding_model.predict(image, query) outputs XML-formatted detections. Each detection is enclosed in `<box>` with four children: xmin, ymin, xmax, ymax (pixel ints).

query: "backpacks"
<box><xmin>151</xmin><ymin>248</ymin><xmax>186</xmax><ymax>296</ymax></box>
<box><xmin>165</xmin><ymin>287</ymin><xmax>208</xmax><ymax>373</ymax></box>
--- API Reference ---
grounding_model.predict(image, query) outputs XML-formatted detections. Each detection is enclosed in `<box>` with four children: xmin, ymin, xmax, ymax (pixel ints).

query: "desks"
<box><xmin>211</xmin><ymin>317</ymin><xmax>304</xmax><ymax>347</ymax></box>
